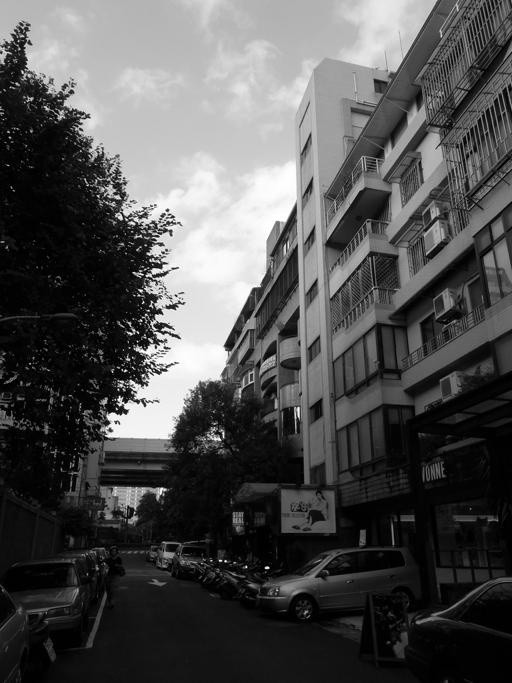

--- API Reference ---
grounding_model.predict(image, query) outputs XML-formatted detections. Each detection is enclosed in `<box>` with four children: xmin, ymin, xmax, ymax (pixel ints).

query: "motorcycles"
<box><xmin>190</xmin><ymin>553</ymin><xmax>291</xmax><ymax>610</ymax></box>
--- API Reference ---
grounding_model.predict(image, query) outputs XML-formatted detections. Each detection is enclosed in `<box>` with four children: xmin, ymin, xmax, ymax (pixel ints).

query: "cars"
<box><xmin>404</xmin><ymin>576</ymin><xmax>511</xmax><ymax>683</ymax></box>
<box><xmin>1</xmin><ymin>547</ymin><xmax>112</xmax><ymax>682</ymax></box>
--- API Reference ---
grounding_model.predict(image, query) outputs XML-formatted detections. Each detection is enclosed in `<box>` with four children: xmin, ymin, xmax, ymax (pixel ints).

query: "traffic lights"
<box><xmin>128</xmin><ymin>507</ymin><xmax>134</xmax><ymax>517</ymax></box>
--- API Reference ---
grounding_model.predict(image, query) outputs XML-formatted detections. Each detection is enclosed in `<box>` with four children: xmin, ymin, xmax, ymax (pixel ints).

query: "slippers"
<box><xmin>302</xmin><ymin>527</ymin><xmax>312</xmax><ymax>530</ymax></box>
<box><xmin>292</xmin><ymin>525</ymin><xmax>300</xmax><ymax>529</ymax></box>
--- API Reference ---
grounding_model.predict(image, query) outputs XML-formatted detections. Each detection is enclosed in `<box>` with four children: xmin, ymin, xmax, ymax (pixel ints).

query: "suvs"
<box><xmin>145</xmin><ymin>540</ymin><xmax>213</xmax><ymax>580</ymax></box>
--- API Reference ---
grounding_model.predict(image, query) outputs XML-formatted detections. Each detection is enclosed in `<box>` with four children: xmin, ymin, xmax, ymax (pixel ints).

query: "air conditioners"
<box><xmin>439</xmin><ymin>371</ymin><xmax>464</xmax><ymax>404</ymax></box>
<box><xmin>432</xmin><ymin>288</ymin><xmax>461</xmax><ymax>324</ymax></box>
<box><xmin>422</xmin><ymin>200</ymin><xmax>452</xmax><ymax>259</ymax></box>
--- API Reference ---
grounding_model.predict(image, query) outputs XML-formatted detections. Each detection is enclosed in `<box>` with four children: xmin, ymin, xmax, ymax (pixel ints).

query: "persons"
<box><xmin>292</xmin><ymin>490</ymin><xmax>328</xmax><ymax>532</ymax></box>
<box><xmin>101</xmin><ymin>546</ymin><xmax>123</xmax><ymax>608</ymax></box>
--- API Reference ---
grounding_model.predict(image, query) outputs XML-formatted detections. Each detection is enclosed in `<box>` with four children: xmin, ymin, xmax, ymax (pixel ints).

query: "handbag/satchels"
<box><xmin>119</xmin><ymin>567</ymin><xmax>126</xmax><ymax>577</ymax></box>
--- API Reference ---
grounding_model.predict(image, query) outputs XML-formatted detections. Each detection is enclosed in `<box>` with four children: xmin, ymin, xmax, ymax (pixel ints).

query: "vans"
<box><xmin>255</xmin><ymin>546</ymin><xmax>422</xmax><ymax>624</ymax></box>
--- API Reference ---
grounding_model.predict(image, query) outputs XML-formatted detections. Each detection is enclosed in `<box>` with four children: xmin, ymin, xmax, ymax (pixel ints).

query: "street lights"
<box><xmin>0</xmin><ymin>310</ymin><xmax>85</xmax><ymax>332</ymax></box>
